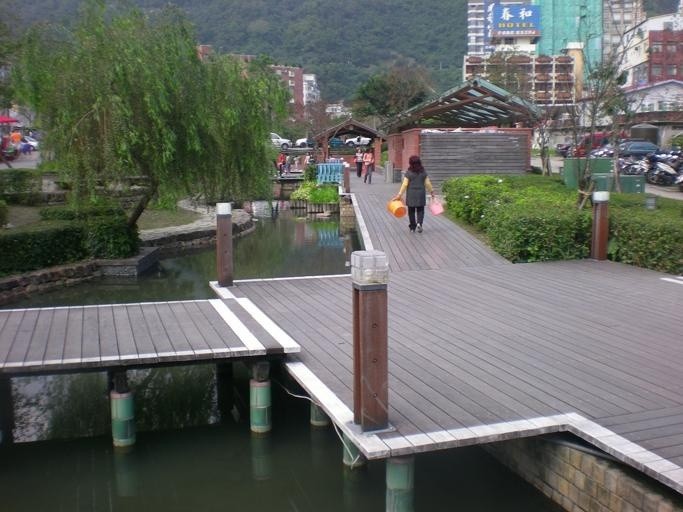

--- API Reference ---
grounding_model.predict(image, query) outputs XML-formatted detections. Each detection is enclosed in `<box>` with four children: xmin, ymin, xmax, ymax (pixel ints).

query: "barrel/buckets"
<box><xmin>387</xmin><ymin>197</ymin><xmax>406</xmax><ymax>217</ymax></box>
<box><xmin>427</xmin><ymin>197</ymin><xmax>444</xmax><ymax>216</ymax></box>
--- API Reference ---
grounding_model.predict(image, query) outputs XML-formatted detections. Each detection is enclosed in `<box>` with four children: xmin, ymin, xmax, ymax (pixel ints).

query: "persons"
<box><xmin>391</xmin><ymin>155</ymin><xmax>435</xmax><ymax>233</ymax></box>
<box><xmin>275</xmin><ymin>151</ymin><xmax>312</xmax><ymax>178</ymax></box>
<box><xmin>361</xmin><ymin>148</ymin><xmax>375</xmax><ymax>184</ymax></box>
<box><xmin>351</xmin><ymin>149</ymin><xmax>363</xmax><ymax>178</ymax></box>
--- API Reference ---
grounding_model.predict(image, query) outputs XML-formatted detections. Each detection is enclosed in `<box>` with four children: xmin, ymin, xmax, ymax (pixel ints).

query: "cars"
<box><xmin>21</xmin><ymin>135</ymin><xmax>39</xmax><ymax>151</ymax></box>
<box><xmin>554</xmin><ymin>129</ymin><xmax>664</xmax><ymax>160</ymax></box>
<box><xmin>269</xmin><ymin>132</ymin><xmax>371</xmax><ymax>151</ymax></box>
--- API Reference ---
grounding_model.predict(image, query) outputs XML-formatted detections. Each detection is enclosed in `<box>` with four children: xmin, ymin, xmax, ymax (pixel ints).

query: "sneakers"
<box><xmin>416</xmin><ymin>222</ymin><xmax>423</xmax><ymax>234</ymax></box>
<box><xmin>410</xmin><ymin>229</ymin><xmax>414</xmax><ymax>232</ymax></box>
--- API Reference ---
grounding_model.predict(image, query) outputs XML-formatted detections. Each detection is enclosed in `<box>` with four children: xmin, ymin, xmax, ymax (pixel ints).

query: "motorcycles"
<box><xmin>615</xmin><ymin>147</ymin><xmax>683</xmax><ymax>192</ymax></box>
